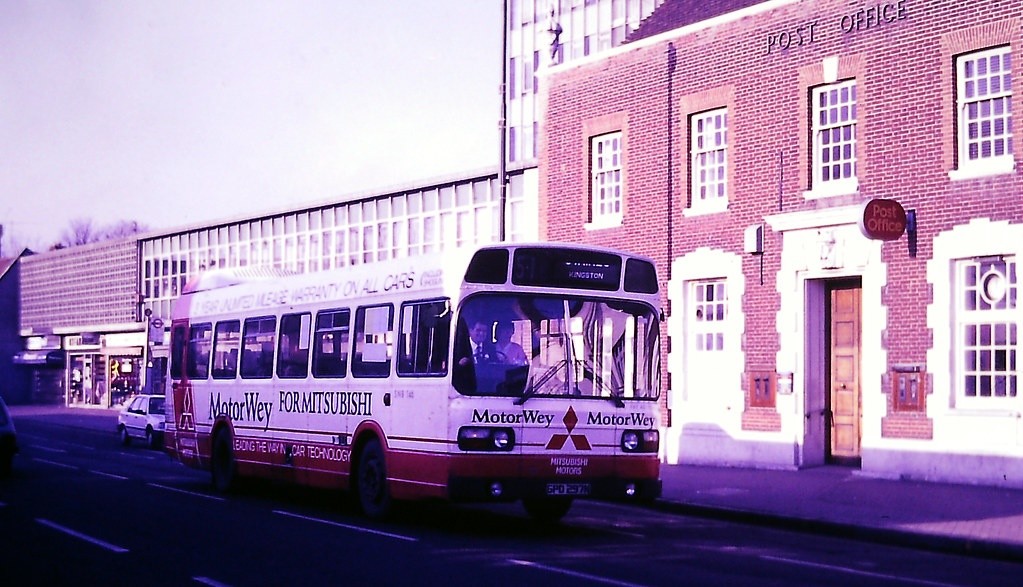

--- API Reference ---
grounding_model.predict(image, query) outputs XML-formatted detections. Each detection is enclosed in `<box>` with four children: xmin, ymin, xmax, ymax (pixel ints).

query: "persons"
<box><xmin>455</xmin><ymin>321</ymin><xmax>501</xmax><ymax>370</ymax></box>
<box><xmin>494</xmin><ymin>321</ymin><xmax>526</xmax><ymax>364</ymax></box>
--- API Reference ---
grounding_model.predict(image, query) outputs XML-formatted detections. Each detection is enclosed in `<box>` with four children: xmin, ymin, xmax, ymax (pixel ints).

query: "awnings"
<box><xmin>13</xmin><ymin>349</ymin><xmax>64</xmax><ymax>364</ymax></box>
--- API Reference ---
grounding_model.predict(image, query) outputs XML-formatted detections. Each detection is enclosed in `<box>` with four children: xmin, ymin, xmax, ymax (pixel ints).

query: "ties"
<box><xmin>476</xmin><ymin>345</ymin><xmax>481</xmax><ymax>363</ymax></box>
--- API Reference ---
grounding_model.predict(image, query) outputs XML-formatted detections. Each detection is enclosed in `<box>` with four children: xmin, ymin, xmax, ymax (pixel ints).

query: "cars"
<box><xmin>116</xmin><ymin>395</ymin><xmax>165</xmax><ymax>447</ymax></box>
<box><xmin>0</xmin><ymin>398</ymin><xmax>18</xmax><ymax>470</ymax></box>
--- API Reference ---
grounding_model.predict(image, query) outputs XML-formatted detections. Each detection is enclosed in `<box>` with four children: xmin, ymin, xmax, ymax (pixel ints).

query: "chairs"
<box><xmin>225</xmin><ymin>348</ymin><xmax>363</xmax><ymax>378</ymax></box>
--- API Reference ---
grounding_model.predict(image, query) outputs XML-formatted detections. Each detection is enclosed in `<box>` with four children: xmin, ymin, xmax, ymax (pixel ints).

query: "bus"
<box><xmin>161</xmin><ymin>241</ymin><xmax>665</xmax><ymax>526</ymax></box>
